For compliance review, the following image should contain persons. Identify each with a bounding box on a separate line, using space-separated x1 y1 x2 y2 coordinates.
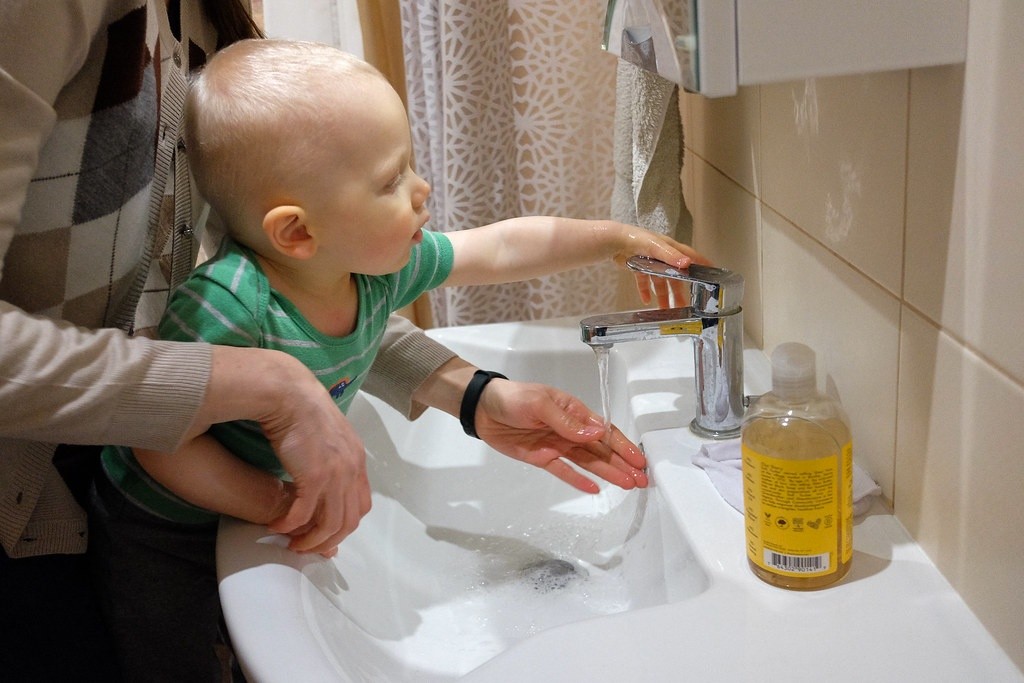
0 0 648 683
86 37 713 683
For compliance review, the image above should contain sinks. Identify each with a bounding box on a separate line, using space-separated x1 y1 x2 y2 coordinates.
213 315 1022 683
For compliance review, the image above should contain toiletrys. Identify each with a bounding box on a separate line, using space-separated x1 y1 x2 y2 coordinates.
740 342 854 591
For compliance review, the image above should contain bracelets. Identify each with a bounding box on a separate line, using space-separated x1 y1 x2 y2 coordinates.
459 369 509 441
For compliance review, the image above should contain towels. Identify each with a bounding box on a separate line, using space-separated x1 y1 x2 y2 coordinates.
599 0 694 247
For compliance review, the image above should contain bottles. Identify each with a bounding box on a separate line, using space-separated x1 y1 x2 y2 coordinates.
741 343 854 590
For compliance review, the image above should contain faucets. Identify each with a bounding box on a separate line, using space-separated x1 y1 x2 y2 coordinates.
580 256 746 438
